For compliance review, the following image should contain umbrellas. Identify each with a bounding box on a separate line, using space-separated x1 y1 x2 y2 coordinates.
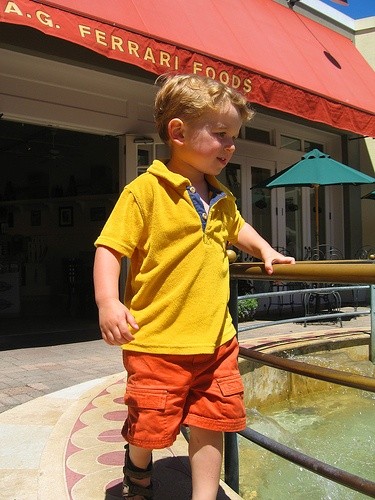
262 148 375 260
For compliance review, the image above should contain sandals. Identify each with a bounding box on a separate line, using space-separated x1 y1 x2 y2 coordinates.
122 444 154 500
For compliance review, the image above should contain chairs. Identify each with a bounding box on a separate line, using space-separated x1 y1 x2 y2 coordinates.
264 244 375 328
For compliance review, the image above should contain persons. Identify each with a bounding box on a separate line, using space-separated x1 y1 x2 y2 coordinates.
92 72 295 500
